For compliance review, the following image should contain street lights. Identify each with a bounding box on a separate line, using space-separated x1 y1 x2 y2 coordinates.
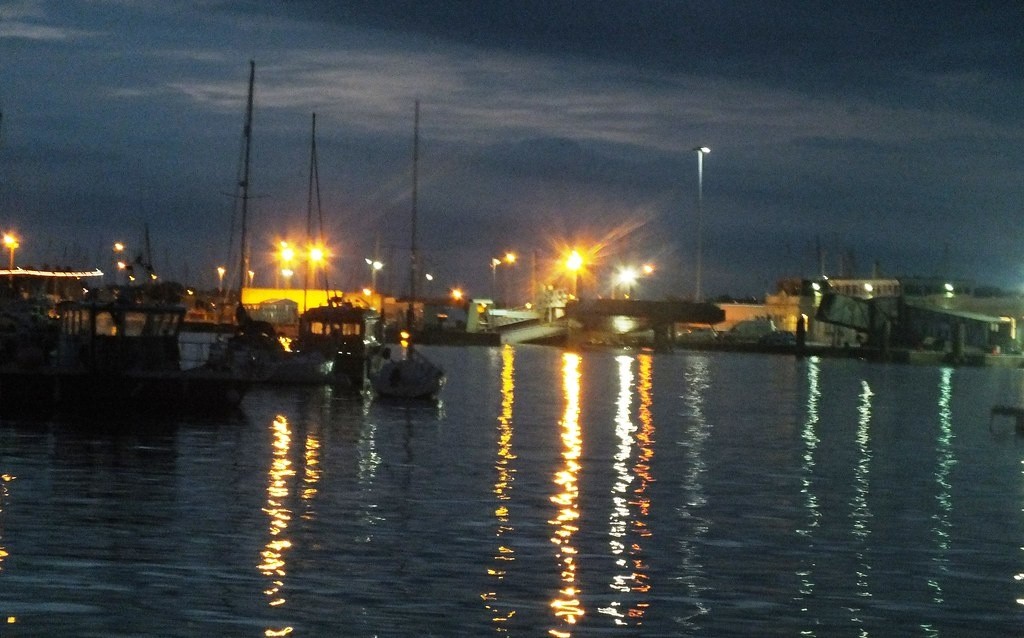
691 145 711 302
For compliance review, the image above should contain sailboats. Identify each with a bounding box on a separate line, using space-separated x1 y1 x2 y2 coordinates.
370 89 448 401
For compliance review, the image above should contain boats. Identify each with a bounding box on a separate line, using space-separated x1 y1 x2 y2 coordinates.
291 296 388 364
